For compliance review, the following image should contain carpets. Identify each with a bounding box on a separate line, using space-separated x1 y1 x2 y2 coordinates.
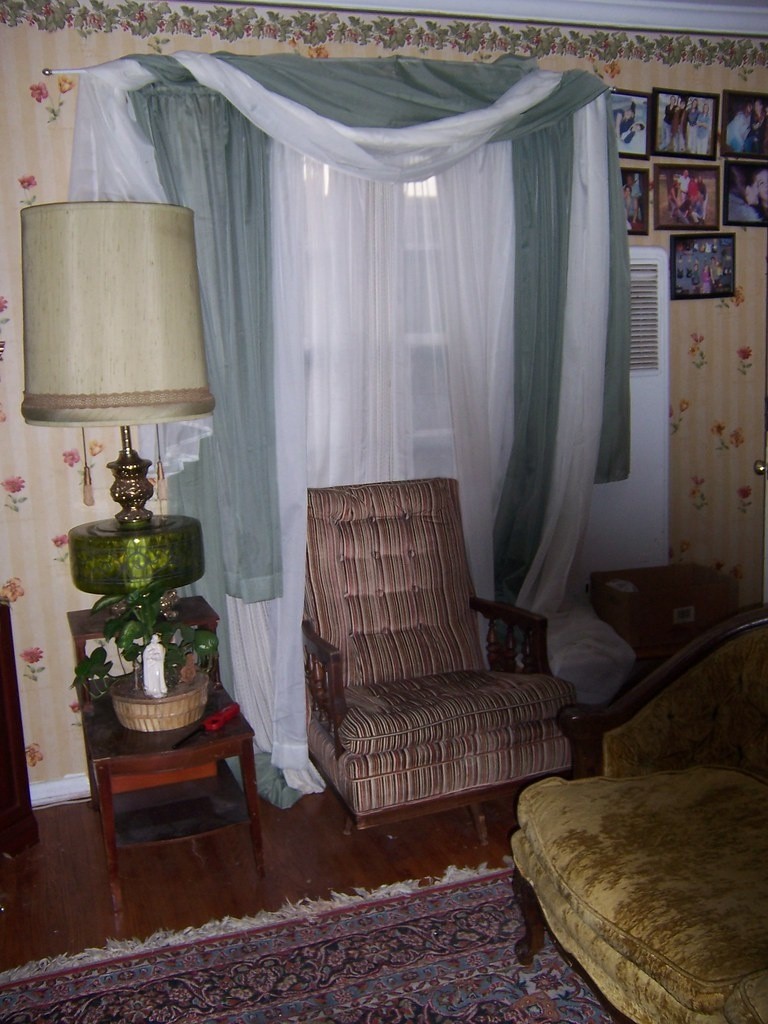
0 862 618 1024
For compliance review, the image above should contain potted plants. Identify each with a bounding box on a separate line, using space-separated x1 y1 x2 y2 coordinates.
72 571 219 733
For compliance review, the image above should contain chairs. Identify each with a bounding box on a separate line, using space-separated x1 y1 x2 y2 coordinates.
302 478 576 846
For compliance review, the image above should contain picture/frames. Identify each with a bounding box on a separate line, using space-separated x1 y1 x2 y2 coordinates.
669 232 736 300
608 86 768 236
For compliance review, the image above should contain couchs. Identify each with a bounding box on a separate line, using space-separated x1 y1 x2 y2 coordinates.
511 606 768 1024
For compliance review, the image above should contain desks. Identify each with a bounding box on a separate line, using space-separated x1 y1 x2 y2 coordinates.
63 596 265 911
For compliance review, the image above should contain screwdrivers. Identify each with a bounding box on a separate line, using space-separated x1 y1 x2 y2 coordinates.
170 701 241 751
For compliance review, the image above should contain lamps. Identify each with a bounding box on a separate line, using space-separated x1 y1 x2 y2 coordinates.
20 201 216 619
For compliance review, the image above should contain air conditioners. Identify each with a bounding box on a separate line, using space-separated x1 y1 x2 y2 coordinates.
581 245 669 579
